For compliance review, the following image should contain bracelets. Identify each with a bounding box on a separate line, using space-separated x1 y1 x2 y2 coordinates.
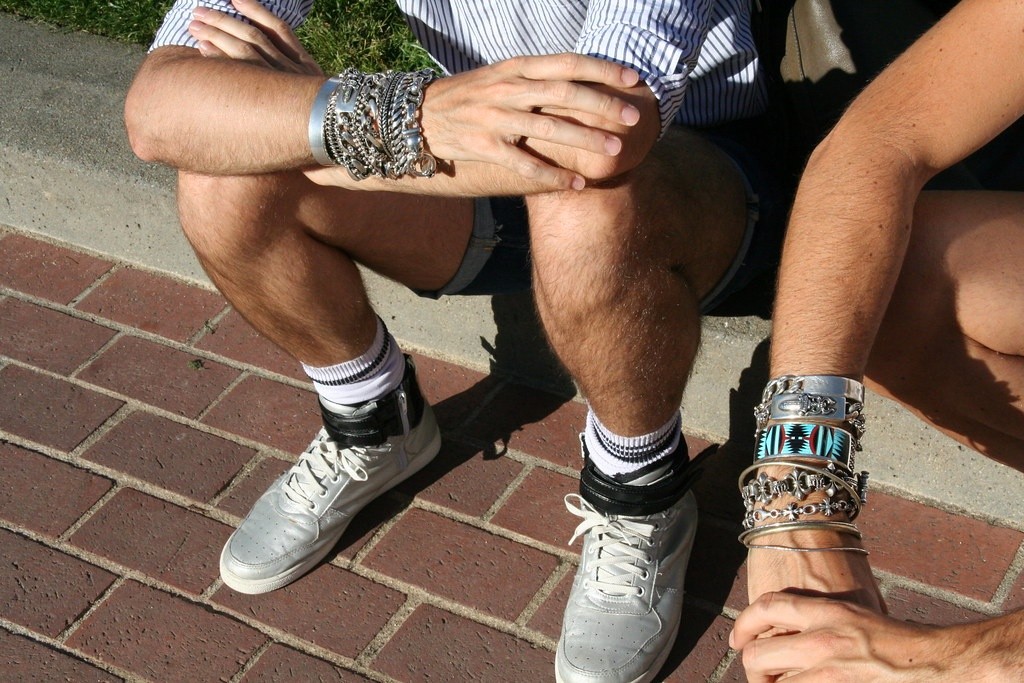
735 374 874 561
306 65 443 182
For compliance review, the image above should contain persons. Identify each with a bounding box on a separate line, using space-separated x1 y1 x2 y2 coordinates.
122 0 815 682
737 0 1023 682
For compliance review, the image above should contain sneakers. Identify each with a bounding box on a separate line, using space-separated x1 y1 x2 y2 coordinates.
220 350 442 595
554 427 719 683
